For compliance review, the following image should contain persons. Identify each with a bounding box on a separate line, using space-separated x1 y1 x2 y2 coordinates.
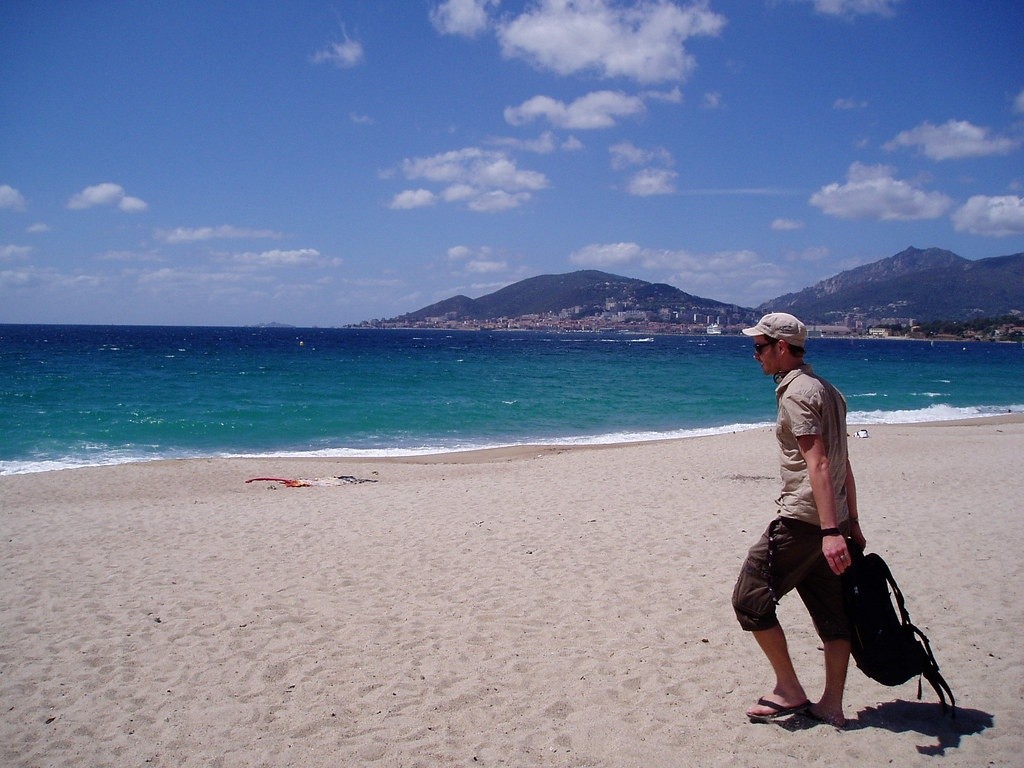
733 313 869 727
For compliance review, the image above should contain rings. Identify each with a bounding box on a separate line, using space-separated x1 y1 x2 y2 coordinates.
840 556 846 559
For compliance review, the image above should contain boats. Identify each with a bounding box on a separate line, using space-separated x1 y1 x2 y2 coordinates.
707 322 722 335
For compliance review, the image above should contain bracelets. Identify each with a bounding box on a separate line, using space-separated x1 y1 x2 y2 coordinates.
821 528 840 537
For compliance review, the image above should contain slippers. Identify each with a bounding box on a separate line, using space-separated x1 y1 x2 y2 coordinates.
793 700 849 729
746 696 810 720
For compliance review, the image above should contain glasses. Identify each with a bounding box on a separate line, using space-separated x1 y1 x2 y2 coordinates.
742 313 806 347
755 341 778 352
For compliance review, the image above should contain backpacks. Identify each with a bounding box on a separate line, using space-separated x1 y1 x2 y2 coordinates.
840 539 939 686
